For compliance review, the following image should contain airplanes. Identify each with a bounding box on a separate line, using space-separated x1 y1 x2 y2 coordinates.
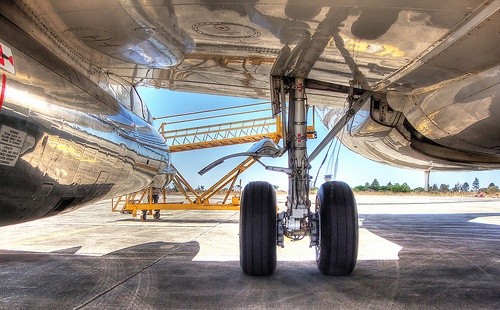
1 0 500 275
